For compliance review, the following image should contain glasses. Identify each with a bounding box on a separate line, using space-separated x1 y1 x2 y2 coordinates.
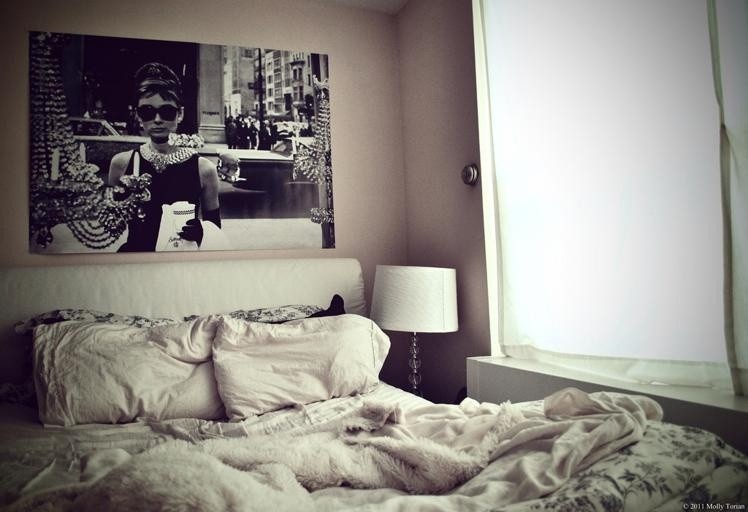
134 104 179 122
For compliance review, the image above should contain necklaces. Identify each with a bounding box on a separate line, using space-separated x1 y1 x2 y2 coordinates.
140 143 200 173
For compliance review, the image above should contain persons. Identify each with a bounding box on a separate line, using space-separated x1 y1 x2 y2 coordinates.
84 101 110 122
224 116 280 152
125 104 141 135
106 62 224 253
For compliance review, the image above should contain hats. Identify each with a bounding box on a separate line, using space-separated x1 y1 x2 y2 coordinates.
134 62 180 87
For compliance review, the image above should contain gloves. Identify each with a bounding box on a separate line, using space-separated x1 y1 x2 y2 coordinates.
178 218 203 242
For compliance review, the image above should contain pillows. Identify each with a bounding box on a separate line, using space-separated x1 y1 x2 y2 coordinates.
13 294 392 427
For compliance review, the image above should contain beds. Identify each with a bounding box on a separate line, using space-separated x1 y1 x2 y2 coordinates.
0 257 748 512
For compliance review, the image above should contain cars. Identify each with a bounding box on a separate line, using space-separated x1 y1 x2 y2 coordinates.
67 117 120 137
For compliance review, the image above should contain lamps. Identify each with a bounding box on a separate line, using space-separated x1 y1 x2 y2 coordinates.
369 264 459 397
